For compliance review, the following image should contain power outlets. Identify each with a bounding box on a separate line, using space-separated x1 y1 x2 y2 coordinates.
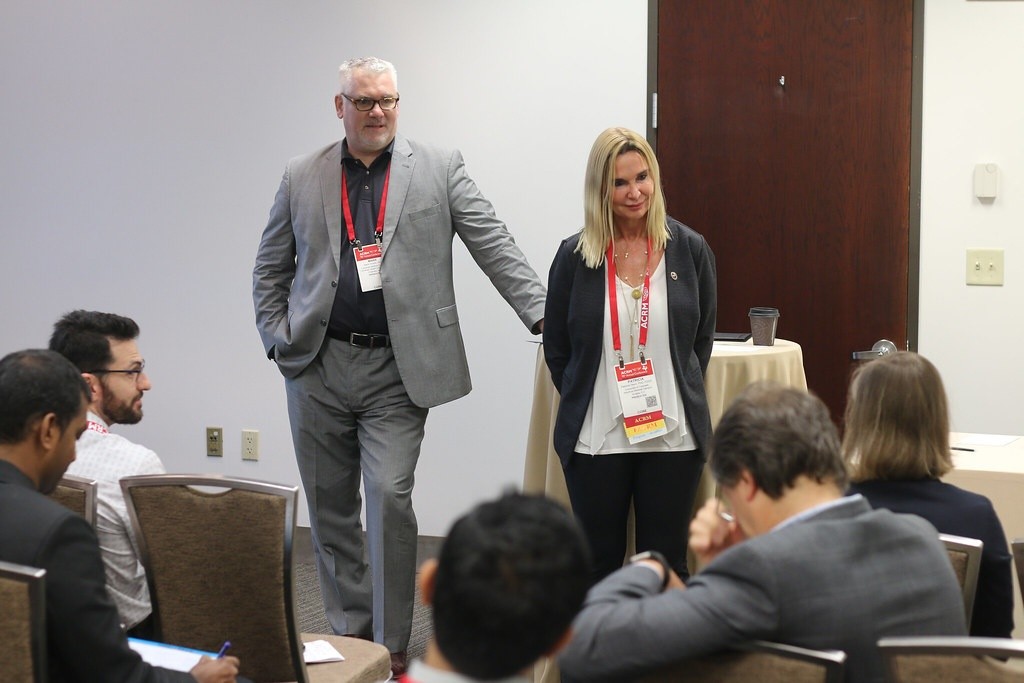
242 431 259 460
206 428 225 456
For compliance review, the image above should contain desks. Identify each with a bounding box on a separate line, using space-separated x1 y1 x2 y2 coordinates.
523 336 808 567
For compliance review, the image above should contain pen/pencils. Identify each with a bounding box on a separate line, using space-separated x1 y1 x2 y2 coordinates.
217 641 231 658
950 447 974 452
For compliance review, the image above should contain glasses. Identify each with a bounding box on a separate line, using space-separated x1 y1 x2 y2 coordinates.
341 92 400 112
87 362 145 383
714 476 742 521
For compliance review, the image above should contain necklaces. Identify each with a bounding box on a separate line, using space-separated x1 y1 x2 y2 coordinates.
614 248 648 336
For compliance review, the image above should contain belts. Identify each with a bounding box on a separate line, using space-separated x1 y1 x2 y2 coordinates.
326 327 391 348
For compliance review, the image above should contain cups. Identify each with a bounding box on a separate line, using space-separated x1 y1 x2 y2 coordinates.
748 306 780 346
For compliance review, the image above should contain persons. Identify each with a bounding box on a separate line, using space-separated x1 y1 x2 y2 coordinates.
49 309 165 635
842 350 1015 662
0 348 242 683
249 58 548 679
393 492 592 683
555 387 967 683
542 127 718 580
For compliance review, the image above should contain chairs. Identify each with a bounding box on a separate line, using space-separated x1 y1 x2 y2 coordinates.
0 470 1024 683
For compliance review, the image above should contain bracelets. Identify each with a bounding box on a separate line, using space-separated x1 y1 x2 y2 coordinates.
628 550 670 591
